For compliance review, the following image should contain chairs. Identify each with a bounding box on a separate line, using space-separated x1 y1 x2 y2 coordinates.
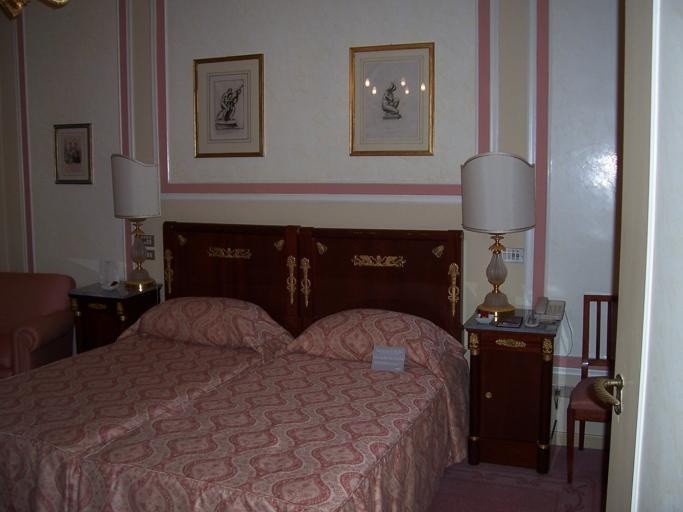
567 292 619 485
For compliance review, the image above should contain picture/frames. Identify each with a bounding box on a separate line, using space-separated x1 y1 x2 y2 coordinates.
54 122 92 185
346 42 434 156
191 54 265 159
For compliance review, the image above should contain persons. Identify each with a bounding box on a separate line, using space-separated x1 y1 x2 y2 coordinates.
216 88 240 120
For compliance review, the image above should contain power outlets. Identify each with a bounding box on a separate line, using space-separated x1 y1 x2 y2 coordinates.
548 384 574 399
152 280 164 293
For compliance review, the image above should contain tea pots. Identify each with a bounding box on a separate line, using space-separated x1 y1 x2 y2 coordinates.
99 259 118 291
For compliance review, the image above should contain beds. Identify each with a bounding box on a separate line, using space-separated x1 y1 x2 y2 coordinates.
3 221 468 512
0 224 300 510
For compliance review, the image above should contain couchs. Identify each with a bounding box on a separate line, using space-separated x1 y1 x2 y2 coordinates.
0 272 77 378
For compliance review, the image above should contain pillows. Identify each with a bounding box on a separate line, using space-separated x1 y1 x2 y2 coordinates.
286 307 465 374
120 294 296 354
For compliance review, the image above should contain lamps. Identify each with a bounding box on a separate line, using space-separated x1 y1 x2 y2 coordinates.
459 152 537 324
112 154 163 290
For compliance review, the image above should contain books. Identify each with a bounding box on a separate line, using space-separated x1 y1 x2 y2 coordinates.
496 315 523 328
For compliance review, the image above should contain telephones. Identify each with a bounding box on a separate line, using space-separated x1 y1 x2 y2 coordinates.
534 297 566 325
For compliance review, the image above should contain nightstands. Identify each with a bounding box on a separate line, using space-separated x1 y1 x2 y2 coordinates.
69 282 159 352
464 308 561 473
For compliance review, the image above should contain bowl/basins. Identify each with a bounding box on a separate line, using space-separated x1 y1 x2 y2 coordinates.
474 313 494 325
